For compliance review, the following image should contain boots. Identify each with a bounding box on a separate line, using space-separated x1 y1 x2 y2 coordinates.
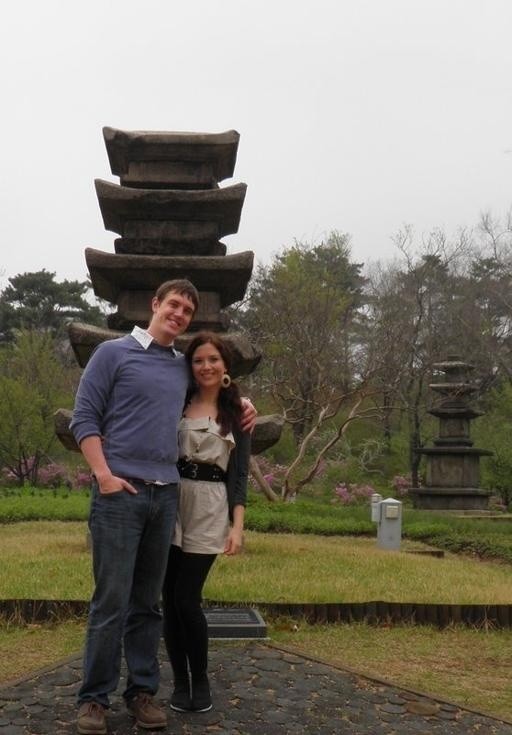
169 657 191 712
189 655 212 713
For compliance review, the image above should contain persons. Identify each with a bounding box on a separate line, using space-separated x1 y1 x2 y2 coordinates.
102 331 250 712
68 278 258 733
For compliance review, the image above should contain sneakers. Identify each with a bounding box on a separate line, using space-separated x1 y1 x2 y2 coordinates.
77 700 106 734
127 694 167 730
